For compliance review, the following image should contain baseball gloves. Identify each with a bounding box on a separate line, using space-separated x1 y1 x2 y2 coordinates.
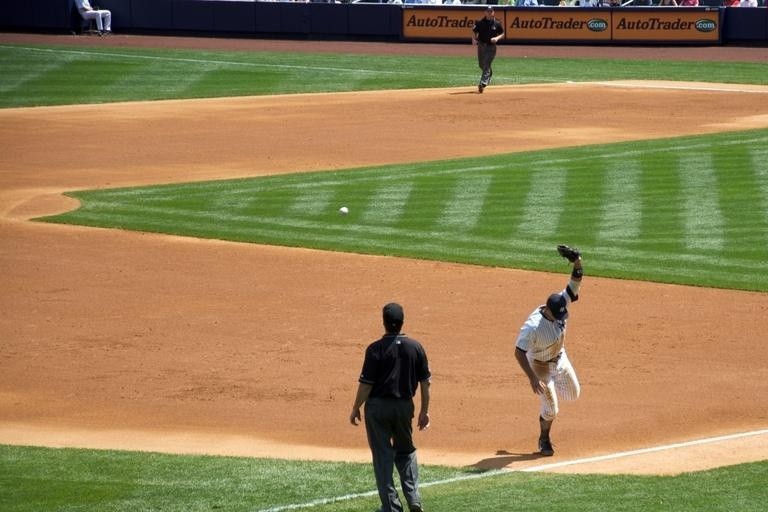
556 244 581 263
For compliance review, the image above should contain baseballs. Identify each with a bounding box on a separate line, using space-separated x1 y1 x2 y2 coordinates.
340 207 350 216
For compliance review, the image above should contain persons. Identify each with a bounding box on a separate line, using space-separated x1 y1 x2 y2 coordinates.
350 302 431 511
516 244 583 457
74 0 113 36
472 7 505 93
386 0 759 7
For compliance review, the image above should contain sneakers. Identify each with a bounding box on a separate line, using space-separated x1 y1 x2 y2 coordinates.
538 437 554 456
409 503 423 512
478 72 491 94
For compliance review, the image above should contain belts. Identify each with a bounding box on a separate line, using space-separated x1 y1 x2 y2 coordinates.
534 352 563 365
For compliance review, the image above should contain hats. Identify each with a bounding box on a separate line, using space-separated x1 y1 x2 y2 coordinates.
382 303 404 331
546 294 569 320
484 5 494 11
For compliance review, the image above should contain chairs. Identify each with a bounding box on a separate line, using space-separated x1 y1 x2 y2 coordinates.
73 0 102 36
